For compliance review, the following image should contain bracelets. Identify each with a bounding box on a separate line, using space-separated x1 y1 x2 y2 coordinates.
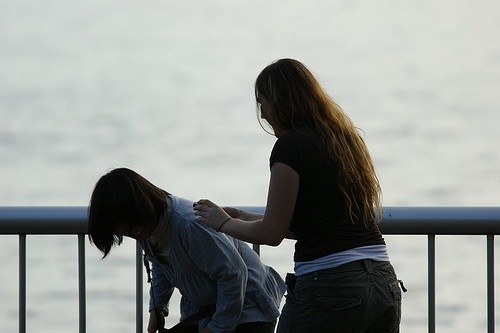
216 218 231 232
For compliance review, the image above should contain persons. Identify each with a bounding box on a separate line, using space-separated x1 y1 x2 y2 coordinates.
192 58 407 332
87 167 287 333
